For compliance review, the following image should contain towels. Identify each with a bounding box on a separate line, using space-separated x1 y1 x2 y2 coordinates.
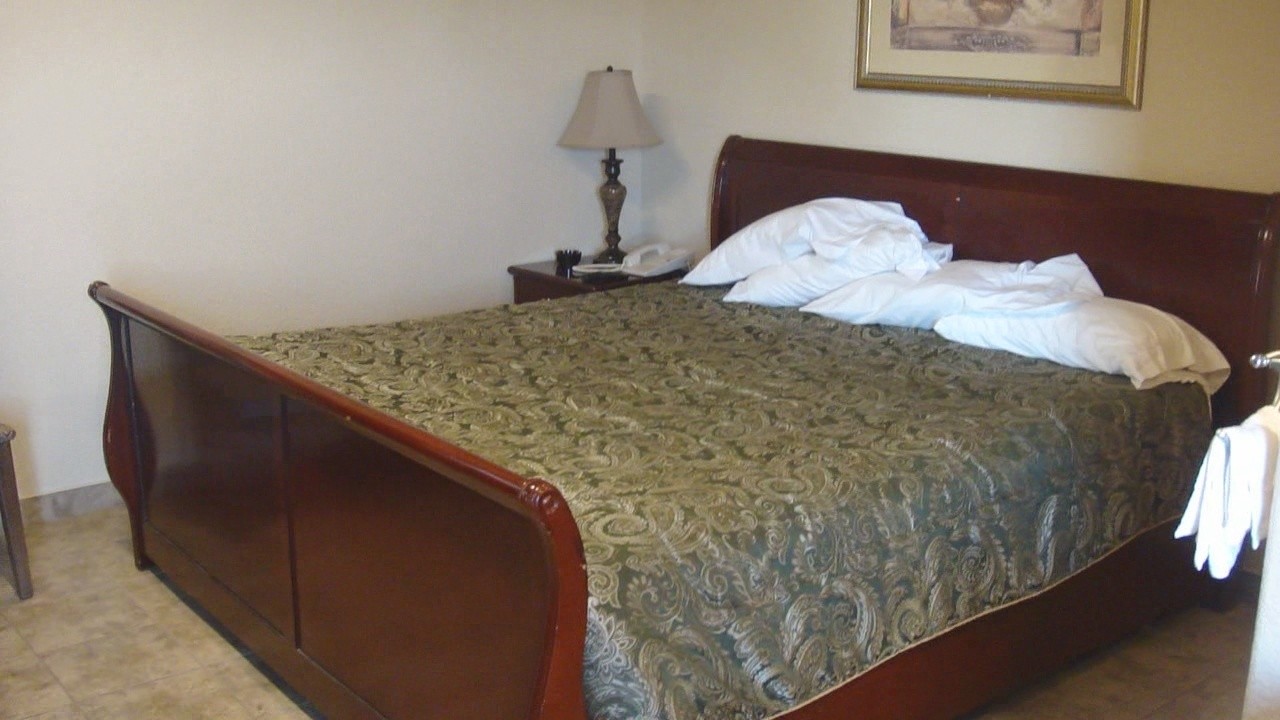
1173 424 1266 580
1243 404 1280 552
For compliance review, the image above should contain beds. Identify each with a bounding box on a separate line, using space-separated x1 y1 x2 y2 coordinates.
86 134 1280 720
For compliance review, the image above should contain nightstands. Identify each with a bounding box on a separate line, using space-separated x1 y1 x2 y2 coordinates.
508 256 684 303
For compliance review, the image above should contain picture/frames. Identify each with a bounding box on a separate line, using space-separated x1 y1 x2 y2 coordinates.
853 0 1151 111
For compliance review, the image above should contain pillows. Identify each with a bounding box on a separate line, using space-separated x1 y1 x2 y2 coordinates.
677 195 1233 394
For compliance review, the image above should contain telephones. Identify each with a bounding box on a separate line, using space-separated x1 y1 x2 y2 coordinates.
620 244 697 278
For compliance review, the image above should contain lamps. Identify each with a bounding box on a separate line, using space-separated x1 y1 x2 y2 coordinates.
555 65 666 263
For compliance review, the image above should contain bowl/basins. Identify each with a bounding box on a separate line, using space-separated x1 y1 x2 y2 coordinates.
557 250 581 267
